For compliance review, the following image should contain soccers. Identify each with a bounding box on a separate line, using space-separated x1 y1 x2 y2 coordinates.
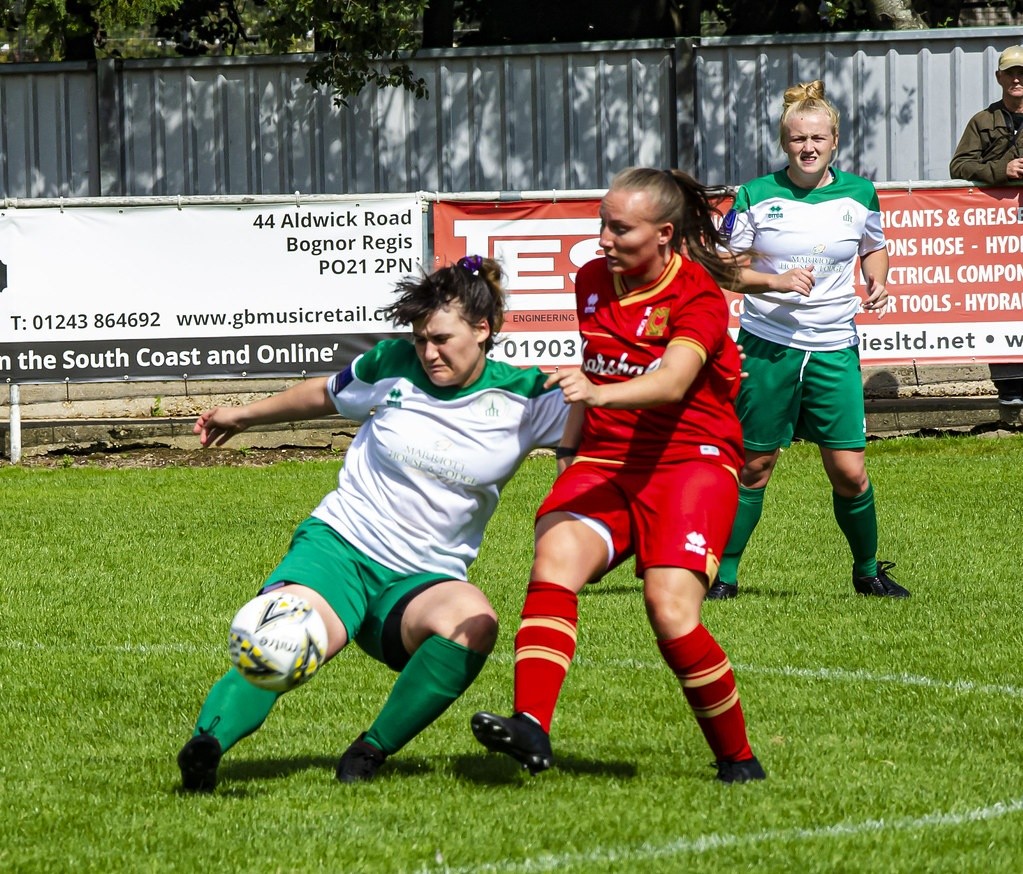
228 591 329 693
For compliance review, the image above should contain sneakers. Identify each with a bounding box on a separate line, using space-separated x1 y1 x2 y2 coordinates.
471 711 553 774
852 560 911 598
336 732 389 786
703 574 738 601
177 715 221 794
710 756 766 785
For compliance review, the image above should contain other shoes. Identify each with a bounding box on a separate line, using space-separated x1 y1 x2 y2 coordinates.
997 389 1023 405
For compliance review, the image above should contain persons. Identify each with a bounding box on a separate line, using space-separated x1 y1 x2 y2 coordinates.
177 258 573 794
710 78 915 598
948 45 1023 432
471 167 767 785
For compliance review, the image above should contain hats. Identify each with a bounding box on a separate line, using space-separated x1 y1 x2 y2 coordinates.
998 44 1023 71
